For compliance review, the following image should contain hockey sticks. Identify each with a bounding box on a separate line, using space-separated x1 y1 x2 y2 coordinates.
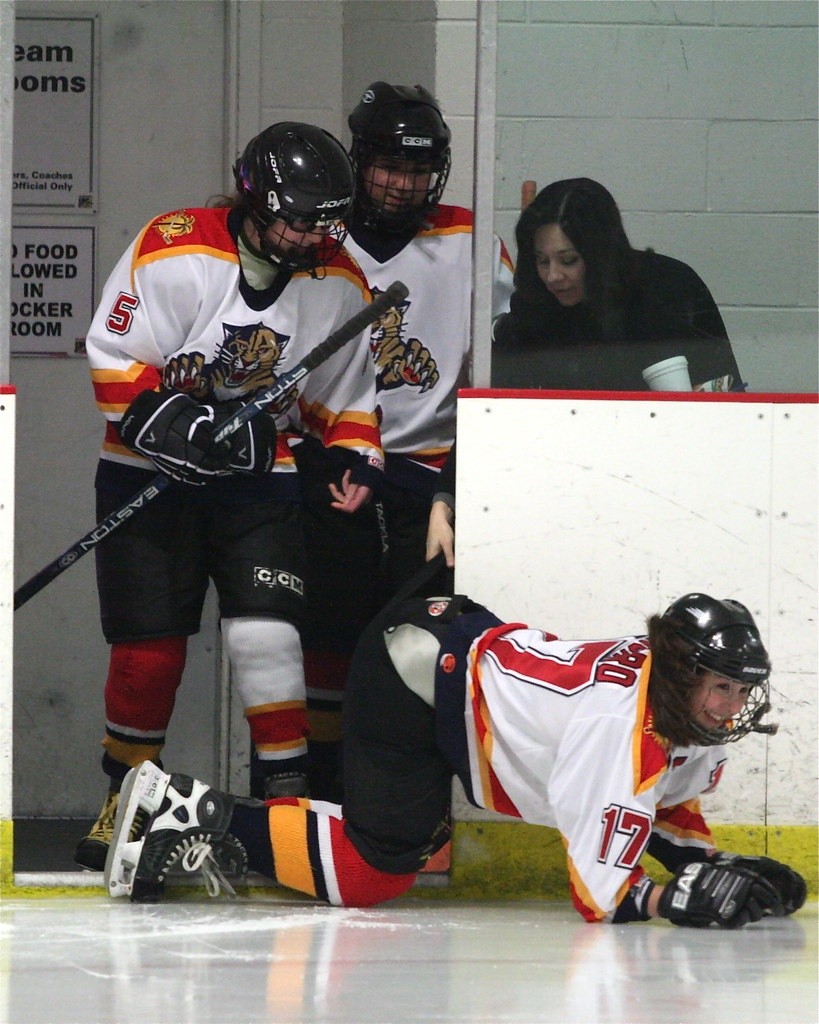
14 275 412 617
515 179 538 269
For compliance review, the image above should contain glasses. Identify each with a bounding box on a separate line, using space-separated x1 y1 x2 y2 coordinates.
265 206 344 233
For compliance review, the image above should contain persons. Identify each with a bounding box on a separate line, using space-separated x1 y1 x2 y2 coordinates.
77 122 385 874
128 593 808 929
426 179 742 599
305 83 515 806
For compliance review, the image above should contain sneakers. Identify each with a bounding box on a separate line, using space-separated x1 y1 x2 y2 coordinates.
103 761 248 901
74 779 125 873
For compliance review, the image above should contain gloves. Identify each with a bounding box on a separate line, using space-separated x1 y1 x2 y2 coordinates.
709 848 807 915
658 863 784 929
116 384 236 488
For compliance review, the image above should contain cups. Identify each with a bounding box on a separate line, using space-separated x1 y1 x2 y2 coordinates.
642 355 693 391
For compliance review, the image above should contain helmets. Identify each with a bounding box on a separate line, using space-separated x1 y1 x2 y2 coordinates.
662 593 771 746
237 123 359 272
348 80 453 228
